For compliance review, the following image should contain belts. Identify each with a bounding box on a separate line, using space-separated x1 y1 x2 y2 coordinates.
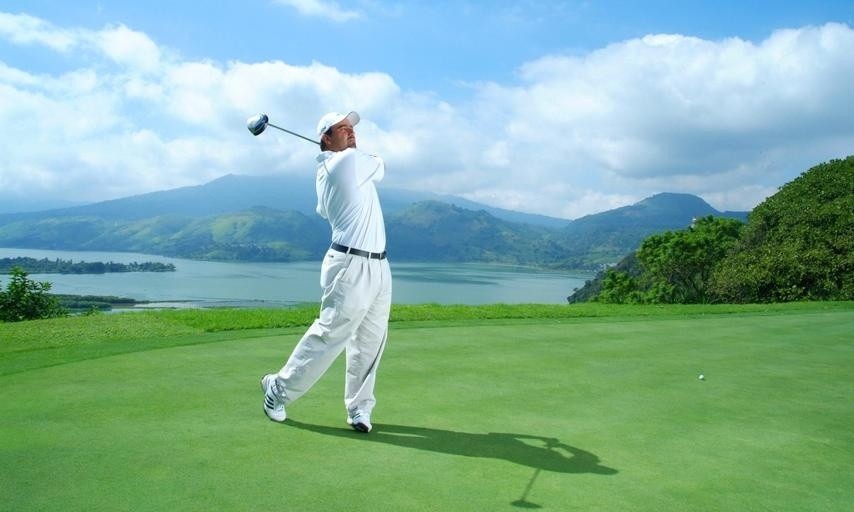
330 243 387 260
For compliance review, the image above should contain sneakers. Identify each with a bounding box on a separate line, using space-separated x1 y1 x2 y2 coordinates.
347 410 373 433
260 373 287 423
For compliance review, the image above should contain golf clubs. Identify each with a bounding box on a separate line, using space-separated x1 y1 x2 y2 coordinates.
247 114 321 146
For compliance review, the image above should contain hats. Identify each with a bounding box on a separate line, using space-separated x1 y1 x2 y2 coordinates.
317 110 361 135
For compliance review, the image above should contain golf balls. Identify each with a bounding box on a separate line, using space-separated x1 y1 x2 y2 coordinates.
699 375 704 379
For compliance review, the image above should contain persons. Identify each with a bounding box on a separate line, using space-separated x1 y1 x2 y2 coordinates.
261 111 393 432
